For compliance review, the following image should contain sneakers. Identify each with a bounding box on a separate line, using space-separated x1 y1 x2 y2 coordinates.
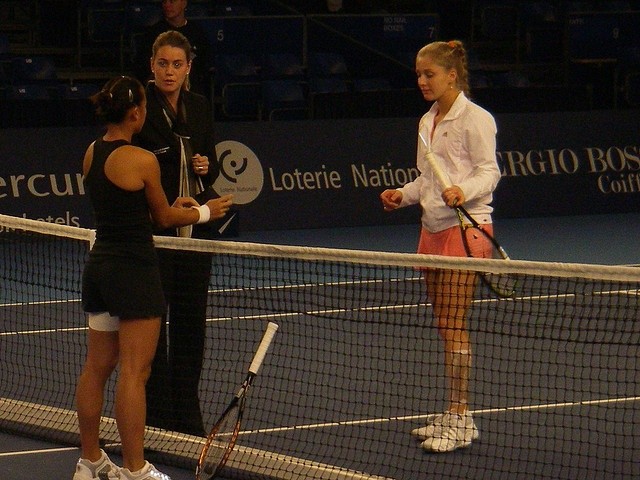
72 448 120 479
107 460 171 480
420 411 471 454
410 410 480 440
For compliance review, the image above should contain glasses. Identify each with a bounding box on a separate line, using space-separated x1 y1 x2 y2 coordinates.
150 58 188 68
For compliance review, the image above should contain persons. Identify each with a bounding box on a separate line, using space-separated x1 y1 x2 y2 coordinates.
141 1 213 76
381 39 501 454
141 28 221 437
74 76 235 480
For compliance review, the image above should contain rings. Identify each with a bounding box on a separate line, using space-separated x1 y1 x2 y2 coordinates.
198 166 203 172
